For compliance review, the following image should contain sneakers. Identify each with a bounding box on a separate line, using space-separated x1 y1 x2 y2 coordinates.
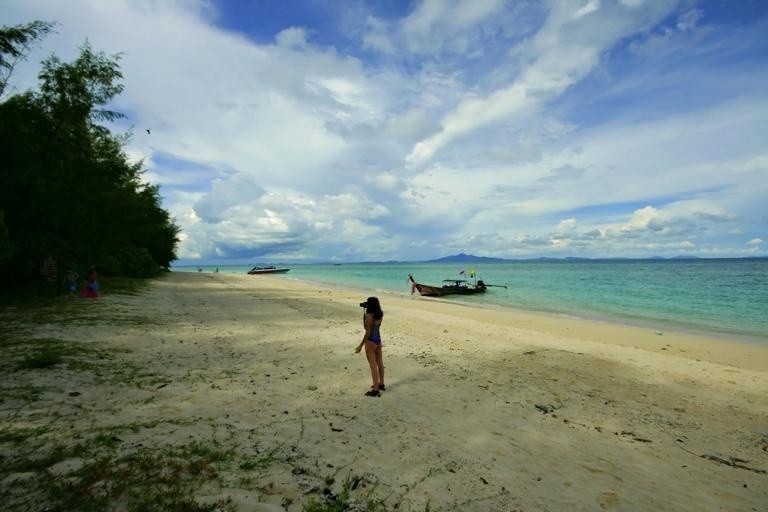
365 390 380 397
371 383 385 391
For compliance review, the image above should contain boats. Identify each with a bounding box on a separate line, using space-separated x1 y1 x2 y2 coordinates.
407 273 487 296
248 265 291 275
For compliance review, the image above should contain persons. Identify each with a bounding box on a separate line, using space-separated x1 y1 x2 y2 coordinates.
354 297 385 397
63 264 79 293
87 264 97 291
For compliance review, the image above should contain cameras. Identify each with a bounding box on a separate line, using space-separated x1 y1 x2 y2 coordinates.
360 302 368 308
